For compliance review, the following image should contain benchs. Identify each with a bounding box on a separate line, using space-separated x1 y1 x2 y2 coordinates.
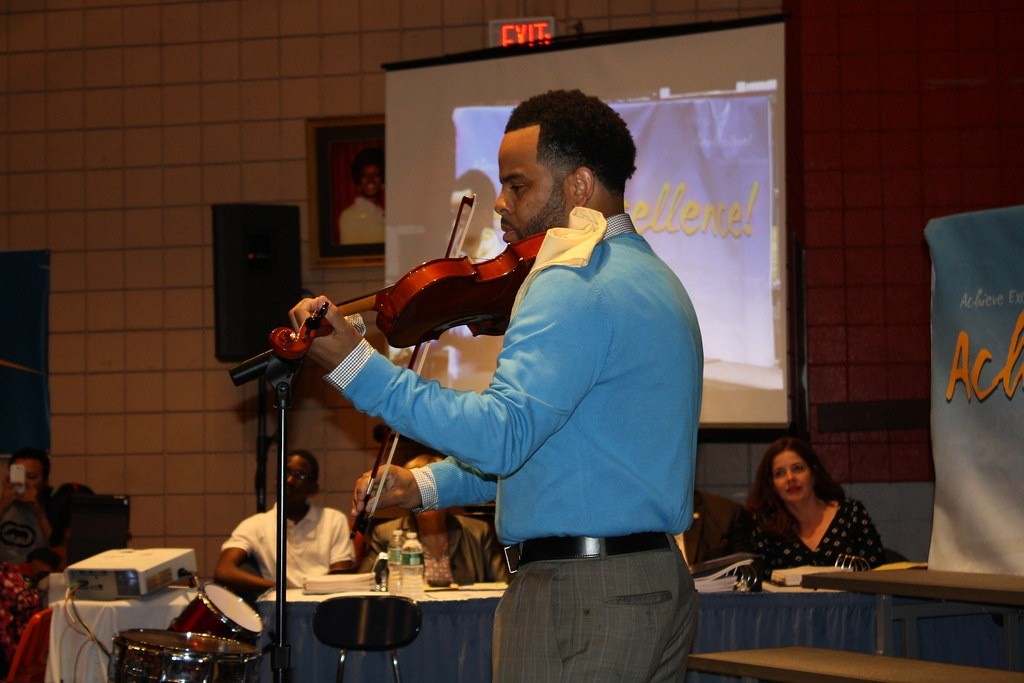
688 646 1024 683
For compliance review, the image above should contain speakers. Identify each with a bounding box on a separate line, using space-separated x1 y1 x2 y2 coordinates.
212 202 303 363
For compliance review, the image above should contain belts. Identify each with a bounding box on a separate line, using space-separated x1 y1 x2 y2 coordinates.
519 532 670 566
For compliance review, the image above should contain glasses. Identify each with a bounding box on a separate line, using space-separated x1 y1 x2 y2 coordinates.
286 470 314 484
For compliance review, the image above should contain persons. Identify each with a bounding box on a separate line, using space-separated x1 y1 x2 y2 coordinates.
218 449 511 602
390 169 512 394
1 450 92 683
290 89 706 682
338 148 385 243
741 436 887 570
674 485 752 580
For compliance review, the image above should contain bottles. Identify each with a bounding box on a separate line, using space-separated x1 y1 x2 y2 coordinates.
386 530 405 594
401 532 424 600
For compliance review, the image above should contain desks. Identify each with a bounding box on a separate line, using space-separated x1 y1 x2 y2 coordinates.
802 568 1024 672
257 580 846 683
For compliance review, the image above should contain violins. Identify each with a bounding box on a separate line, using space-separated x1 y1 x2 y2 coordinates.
268 231 552 362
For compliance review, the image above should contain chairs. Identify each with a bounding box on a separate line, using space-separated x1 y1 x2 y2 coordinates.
6 608 53 683
313 595 420 683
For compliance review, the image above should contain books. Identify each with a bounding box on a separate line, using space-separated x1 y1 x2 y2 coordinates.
694 558 928 593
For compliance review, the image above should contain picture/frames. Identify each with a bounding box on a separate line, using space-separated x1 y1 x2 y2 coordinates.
306 115 385 265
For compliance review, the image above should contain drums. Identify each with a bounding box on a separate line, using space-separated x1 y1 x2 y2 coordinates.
168 581 264 643
106 627 262 683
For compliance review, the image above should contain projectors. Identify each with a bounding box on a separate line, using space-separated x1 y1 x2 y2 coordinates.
65 548 197 601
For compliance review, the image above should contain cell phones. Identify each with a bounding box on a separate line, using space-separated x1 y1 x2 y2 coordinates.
10 464 25 493
